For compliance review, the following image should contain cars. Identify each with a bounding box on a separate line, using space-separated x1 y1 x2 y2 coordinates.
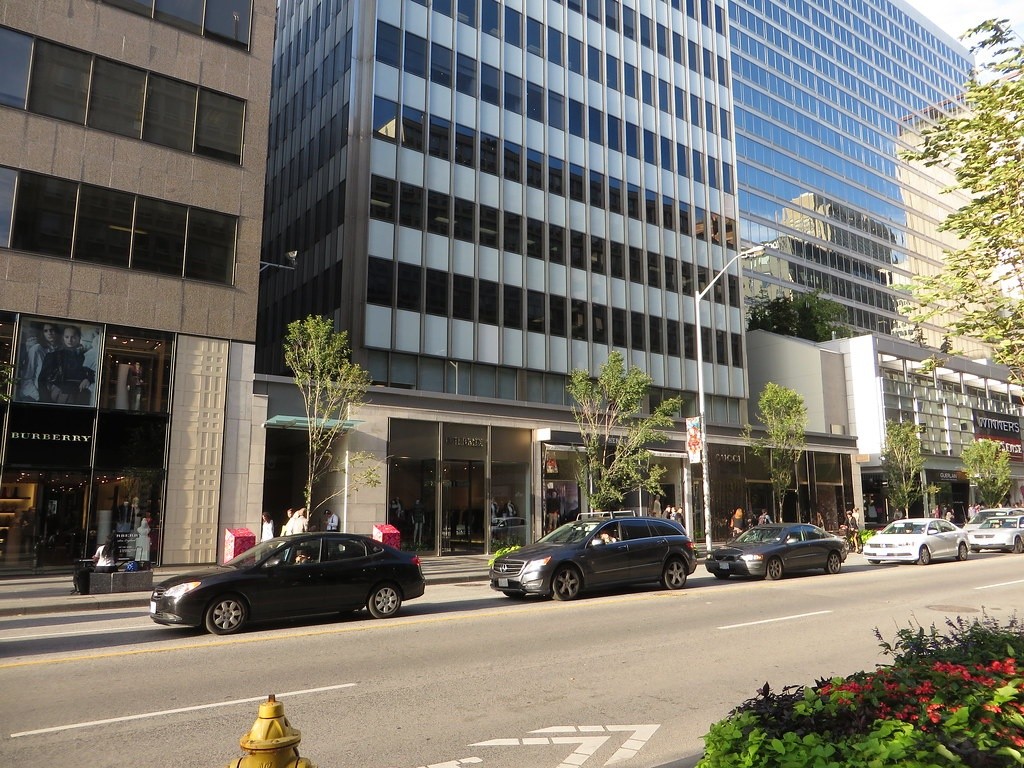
149 532 426 635
490 517 527 538
704 523 847 580
961 508 1024 531
862 518 971 565
490 510 699 601
967 515 1024 554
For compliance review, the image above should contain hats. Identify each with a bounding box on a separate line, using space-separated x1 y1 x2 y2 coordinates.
297 549 315 561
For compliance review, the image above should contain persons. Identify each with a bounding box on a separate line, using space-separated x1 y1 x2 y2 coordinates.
325 509 341 553
92 533 118 573
546 485 561 533
284 508 309 563
491 497 518 547
412 499 426 549
297 548 316 564
893 508 903 520
21 322 93 406
731 508 745 539
601 528 616 544
758 509 773 539
654 495 662 518
127 362 145 412
776 533 797 543
841 507 861 553
968 500 1024 529
934 504 955 524
260 513 273 565
113 497 149 560
664 505 686 528
389 497 401 527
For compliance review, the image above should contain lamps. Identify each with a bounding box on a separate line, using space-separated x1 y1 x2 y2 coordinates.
258 249 298 272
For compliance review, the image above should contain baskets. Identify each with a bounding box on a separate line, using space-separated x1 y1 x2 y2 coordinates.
839 527 848 536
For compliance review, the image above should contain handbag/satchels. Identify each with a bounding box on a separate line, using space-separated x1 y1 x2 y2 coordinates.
764 514 769 524
124 560 138 572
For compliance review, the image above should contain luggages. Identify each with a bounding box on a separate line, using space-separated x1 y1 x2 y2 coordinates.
76 567 94 595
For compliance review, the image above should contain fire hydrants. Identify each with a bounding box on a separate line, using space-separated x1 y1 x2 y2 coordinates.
224 694 319 768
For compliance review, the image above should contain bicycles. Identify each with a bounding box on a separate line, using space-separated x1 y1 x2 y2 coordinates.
842 527 863 554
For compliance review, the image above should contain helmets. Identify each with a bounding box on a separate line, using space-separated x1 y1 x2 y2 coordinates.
846 511 852 515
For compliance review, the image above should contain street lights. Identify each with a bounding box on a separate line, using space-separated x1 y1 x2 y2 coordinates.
695 245 766 559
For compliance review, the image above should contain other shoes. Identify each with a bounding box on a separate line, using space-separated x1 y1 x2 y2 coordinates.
855 547 859 553
